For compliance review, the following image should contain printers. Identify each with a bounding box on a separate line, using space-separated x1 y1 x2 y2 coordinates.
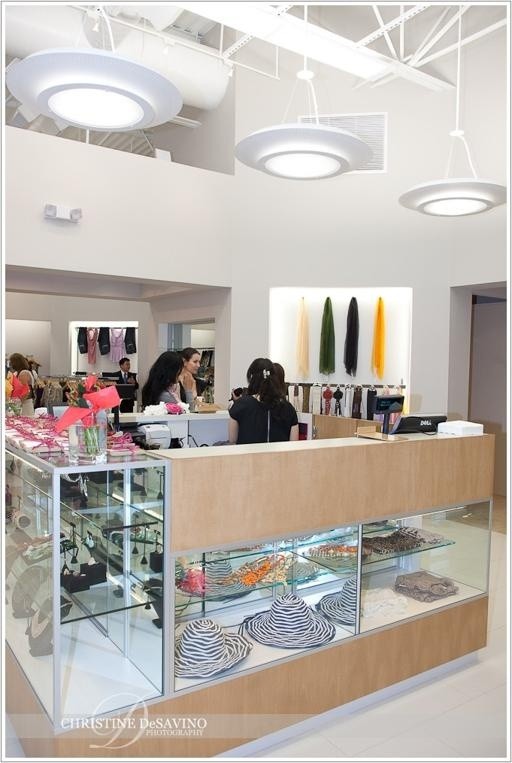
141 423 173 448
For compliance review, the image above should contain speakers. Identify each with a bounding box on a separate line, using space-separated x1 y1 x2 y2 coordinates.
43 203 84 223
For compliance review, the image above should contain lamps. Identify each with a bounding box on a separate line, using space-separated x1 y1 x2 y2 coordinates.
397 5 506 216
6 6 182 132
233 6 372 180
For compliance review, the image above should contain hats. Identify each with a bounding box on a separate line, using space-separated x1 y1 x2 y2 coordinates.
205 557 255 601
278 562 318 584
175 594 336 678
318 576 356 626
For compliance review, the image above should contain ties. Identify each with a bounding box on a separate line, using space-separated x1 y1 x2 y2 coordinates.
124 373 126 384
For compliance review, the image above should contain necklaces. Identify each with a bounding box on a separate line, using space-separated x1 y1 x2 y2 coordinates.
31 602 72 638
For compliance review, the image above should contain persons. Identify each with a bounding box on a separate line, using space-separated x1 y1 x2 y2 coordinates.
228 358 299 444
142 347 201 411
112 358 139 413
11 353 41 399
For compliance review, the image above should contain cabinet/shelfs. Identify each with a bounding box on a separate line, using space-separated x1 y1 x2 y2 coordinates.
5 418 492 758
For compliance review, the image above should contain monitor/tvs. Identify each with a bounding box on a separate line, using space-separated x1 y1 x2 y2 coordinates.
391 413 447 434
